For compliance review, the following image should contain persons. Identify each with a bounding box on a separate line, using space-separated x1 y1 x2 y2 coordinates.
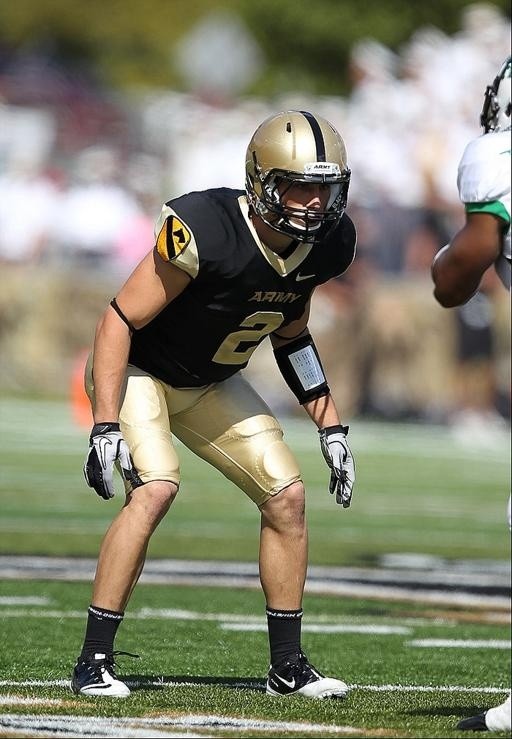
71 111 356 703
427 57 512 734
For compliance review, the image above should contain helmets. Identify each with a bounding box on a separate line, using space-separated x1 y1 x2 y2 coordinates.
244 108 352 243
480 53 512 133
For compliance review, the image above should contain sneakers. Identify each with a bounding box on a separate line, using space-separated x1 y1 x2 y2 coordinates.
70 648 131 700
265 650 351 701
456 693 512 733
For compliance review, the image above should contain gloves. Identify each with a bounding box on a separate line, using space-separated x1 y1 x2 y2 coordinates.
317 424 357 510
81 420 134 501
